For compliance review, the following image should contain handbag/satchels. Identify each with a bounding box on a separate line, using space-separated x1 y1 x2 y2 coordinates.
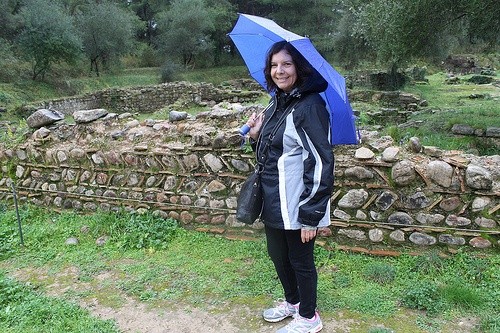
236 171 263 224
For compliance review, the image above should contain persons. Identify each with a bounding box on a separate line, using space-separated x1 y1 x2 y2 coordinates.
245 40 335 333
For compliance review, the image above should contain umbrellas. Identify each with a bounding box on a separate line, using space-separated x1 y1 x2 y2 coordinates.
226 13 361 147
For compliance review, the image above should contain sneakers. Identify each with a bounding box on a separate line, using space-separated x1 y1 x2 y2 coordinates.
276 312 323 333
262 298 300 322
